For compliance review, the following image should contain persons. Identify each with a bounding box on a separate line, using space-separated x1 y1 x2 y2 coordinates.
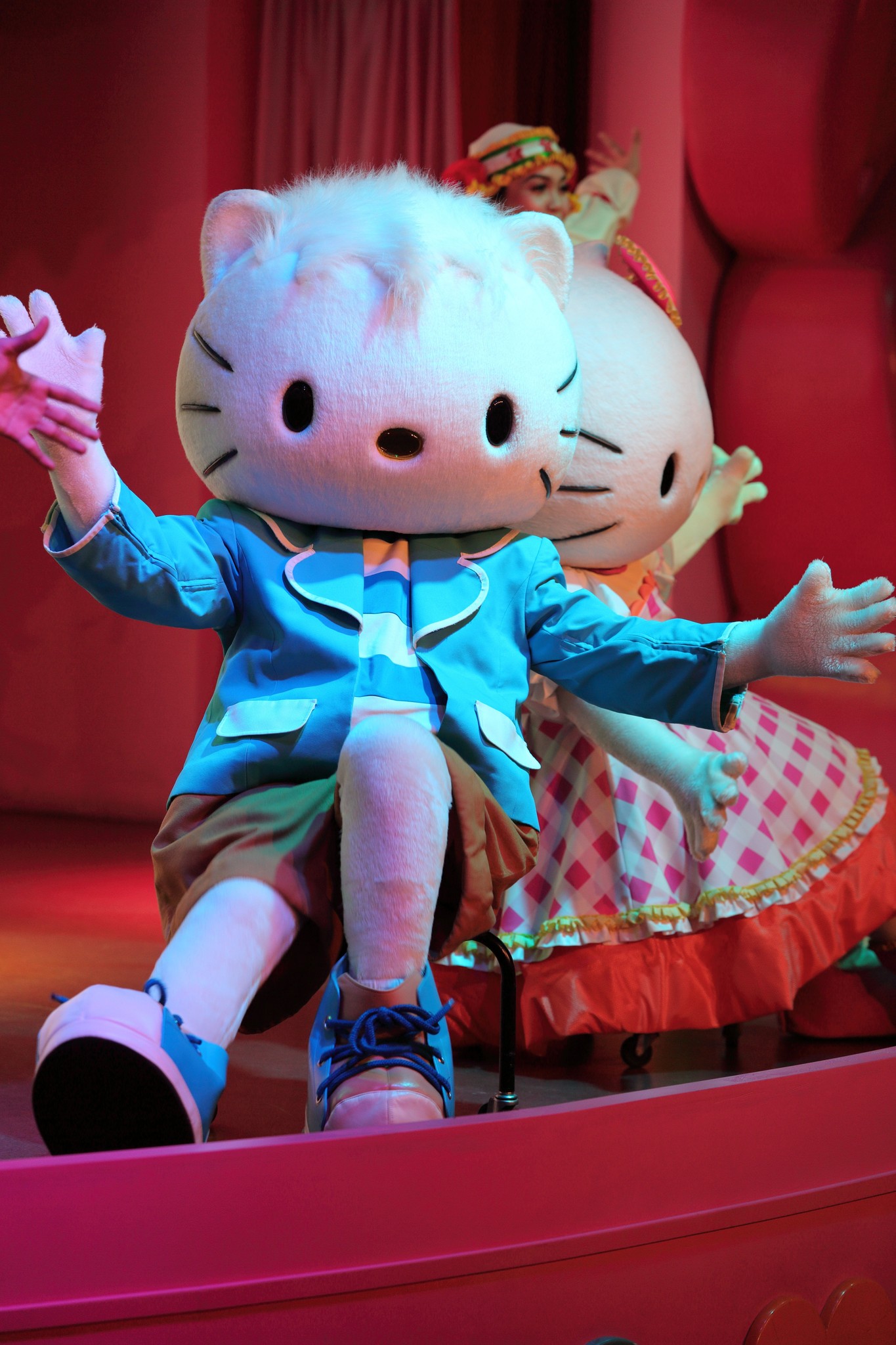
1 316 103 468
441 123 645 267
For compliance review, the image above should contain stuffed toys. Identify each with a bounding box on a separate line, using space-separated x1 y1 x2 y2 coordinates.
436 264 891 1094
0 162 895 1158
